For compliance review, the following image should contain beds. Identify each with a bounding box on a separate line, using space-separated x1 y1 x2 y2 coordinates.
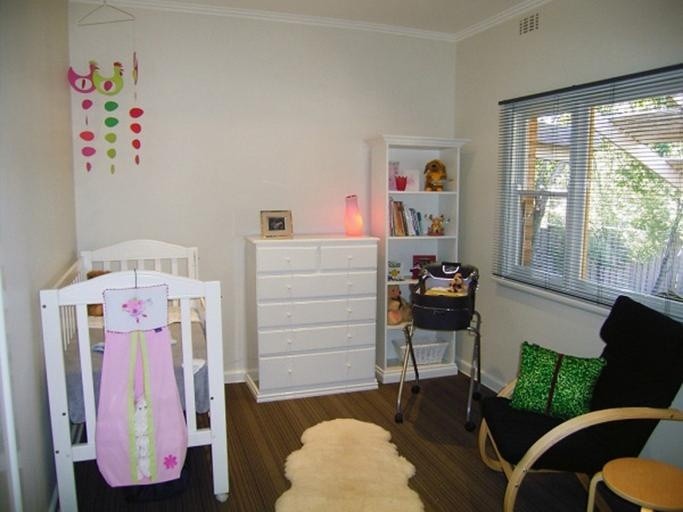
37 237 232 512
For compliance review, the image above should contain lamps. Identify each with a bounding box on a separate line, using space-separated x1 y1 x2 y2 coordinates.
343 194 363 236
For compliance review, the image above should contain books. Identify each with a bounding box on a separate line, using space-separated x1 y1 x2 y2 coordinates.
389 196 424 237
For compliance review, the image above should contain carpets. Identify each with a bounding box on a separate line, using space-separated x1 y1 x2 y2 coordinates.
269 417 427 512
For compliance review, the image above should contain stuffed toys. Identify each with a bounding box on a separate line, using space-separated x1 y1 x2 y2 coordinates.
447 272 467 292
387 285 413 326
422 158 447 192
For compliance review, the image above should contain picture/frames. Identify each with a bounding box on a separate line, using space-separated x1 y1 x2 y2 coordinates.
411 255 436 278
259 208 294 239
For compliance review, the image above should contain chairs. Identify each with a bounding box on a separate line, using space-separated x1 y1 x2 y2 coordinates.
475 294 682 512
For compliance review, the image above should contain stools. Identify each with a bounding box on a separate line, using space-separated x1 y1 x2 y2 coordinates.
585 457 683 512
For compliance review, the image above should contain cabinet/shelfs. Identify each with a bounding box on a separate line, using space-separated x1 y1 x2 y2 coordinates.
364 133 467 387
244 232 379 404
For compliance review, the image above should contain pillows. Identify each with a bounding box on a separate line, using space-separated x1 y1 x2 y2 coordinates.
508 339 607 422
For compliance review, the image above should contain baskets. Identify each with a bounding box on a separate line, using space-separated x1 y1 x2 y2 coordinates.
393 338 450 365
